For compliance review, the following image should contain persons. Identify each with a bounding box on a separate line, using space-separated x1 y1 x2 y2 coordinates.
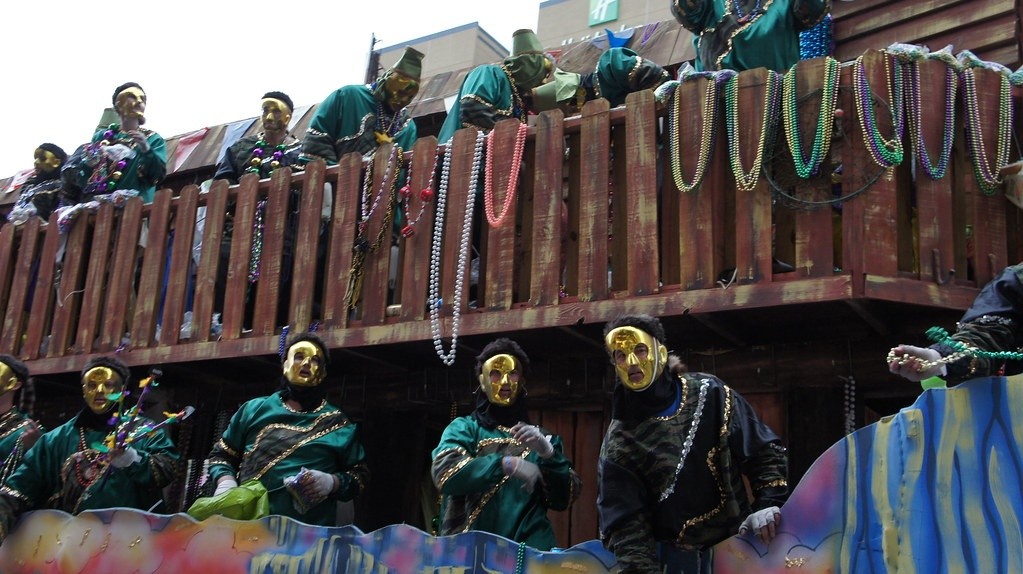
431 337 582 551
529 48 671 114
210 334 374 523
215 89 301 283
887 263 1022 387
669 1 832 73
7 143 68 227
298 47 426 250
0 356 48 488
0 357 185 549
60 81 168 249
437 31 555 198
597 315 789 574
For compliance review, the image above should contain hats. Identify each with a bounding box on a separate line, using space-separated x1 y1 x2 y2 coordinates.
512 29 544 55
394 46 424 83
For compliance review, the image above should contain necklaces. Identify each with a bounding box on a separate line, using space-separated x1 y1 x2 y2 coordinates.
670 47 1013 195
339 120 528 366
247 136 285 177
87 123 128 191
249 199 267 282
0 424 44 488
515 542 525 573
374 93 399 139
77 426 113 487
888 327 1022 370
